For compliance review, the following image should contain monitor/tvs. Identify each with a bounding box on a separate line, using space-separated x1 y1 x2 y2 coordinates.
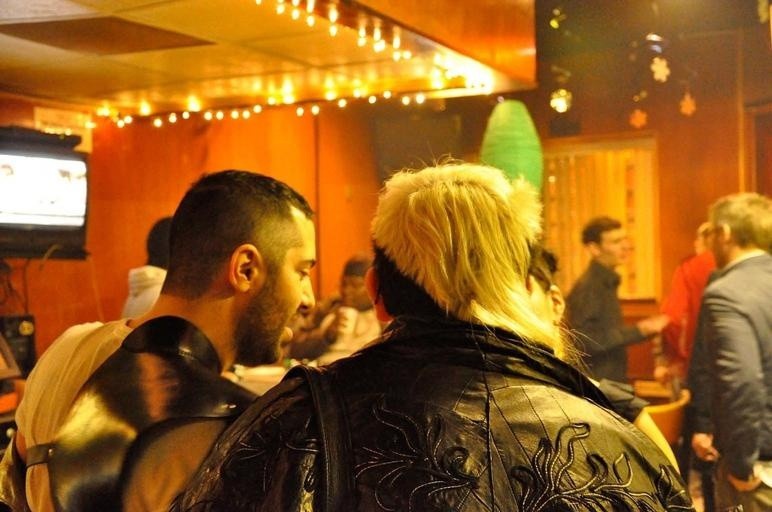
0 139 89 230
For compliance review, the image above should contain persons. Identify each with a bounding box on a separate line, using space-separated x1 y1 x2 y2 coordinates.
1 168 320 512
525 244 567 328
698 187 772 512
283 253 393 369
678 263 731 511
563 216 671 382
513 244 685 495
122 216 174 320
659 221 719 376
165 155 697 512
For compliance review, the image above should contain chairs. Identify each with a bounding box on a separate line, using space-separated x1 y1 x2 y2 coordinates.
645 387 691 478
